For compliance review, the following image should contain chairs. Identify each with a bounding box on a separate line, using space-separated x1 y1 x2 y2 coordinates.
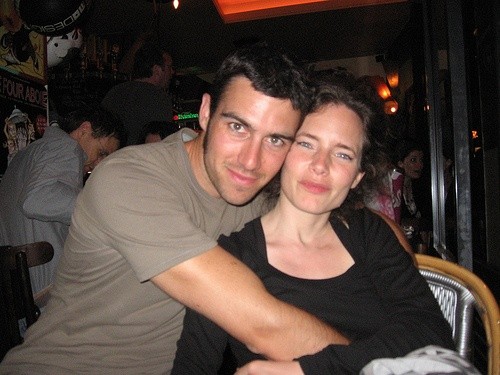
415 253 500 375
0 241 54 364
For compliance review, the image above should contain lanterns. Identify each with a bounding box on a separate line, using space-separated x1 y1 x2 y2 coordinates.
376 71 399 115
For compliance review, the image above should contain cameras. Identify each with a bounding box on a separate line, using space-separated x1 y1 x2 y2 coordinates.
403 226 415 239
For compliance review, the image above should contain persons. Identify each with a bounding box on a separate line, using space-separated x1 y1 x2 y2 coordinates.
0 41 477 337
171 68 459 375
0 43 353 375
